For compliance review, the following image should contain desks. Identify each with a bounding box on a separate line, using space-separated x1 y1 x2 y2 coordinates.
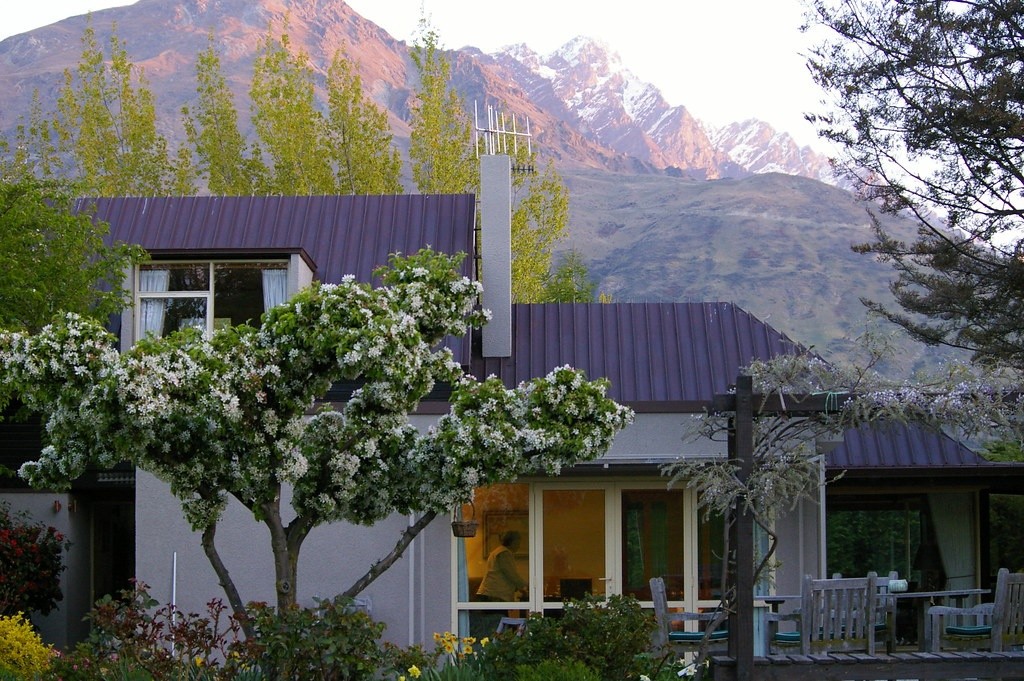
754 588 991 652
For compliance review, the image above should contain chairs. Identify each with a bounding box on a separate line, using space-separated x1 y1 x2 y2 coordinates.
648 571 901 659
927 568 1024 652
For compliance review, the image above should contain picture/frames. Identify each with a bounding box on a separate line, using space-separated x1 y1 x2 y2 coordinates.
482 509 529 559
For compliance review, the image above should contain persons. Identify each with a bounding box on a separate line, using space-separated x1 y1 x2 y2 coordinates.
475 531 528 637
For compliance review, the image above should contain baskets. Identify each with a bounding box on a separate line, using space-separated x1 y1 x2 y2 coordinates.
451 499 480 538
